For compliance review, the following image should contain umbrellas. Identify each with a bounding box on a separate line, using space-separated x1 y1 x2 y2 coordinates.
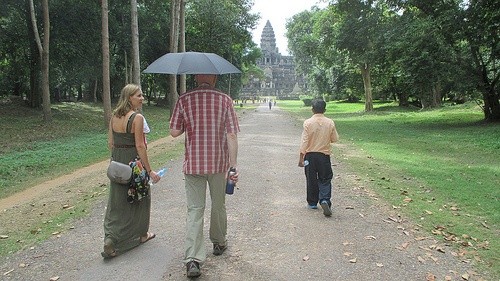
143 50 243 75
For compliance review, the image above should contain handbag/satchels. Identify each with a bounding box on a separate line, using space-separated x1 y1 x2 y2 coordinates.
107 161 133 184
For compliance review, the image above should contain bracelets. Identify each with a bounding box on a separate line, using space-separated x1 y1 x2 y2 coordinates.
147 169 151 172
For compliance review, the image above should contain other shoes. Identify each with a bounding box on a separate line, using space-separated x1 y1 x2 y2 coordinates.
310 204 317 209
213 242 226 255
321 201 331 215
187 261 201 276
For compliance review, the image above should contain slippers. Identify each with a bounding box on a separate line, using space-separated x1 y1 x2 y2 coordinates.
101 244 114 259
139 233 157 243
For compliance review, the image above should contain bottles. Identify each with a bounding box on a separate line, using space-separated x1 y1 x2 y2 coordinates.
150 168 167 183
302 160 309 166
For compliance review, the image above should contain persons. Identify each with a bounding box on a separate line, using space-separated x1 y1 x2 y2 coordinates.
298 99 339 216
169 73 241 277
234 98 277 111
101 84 157 258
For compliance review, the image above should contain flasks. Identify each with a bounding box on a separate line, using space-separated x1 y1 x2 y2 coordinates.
226 166 236 194
